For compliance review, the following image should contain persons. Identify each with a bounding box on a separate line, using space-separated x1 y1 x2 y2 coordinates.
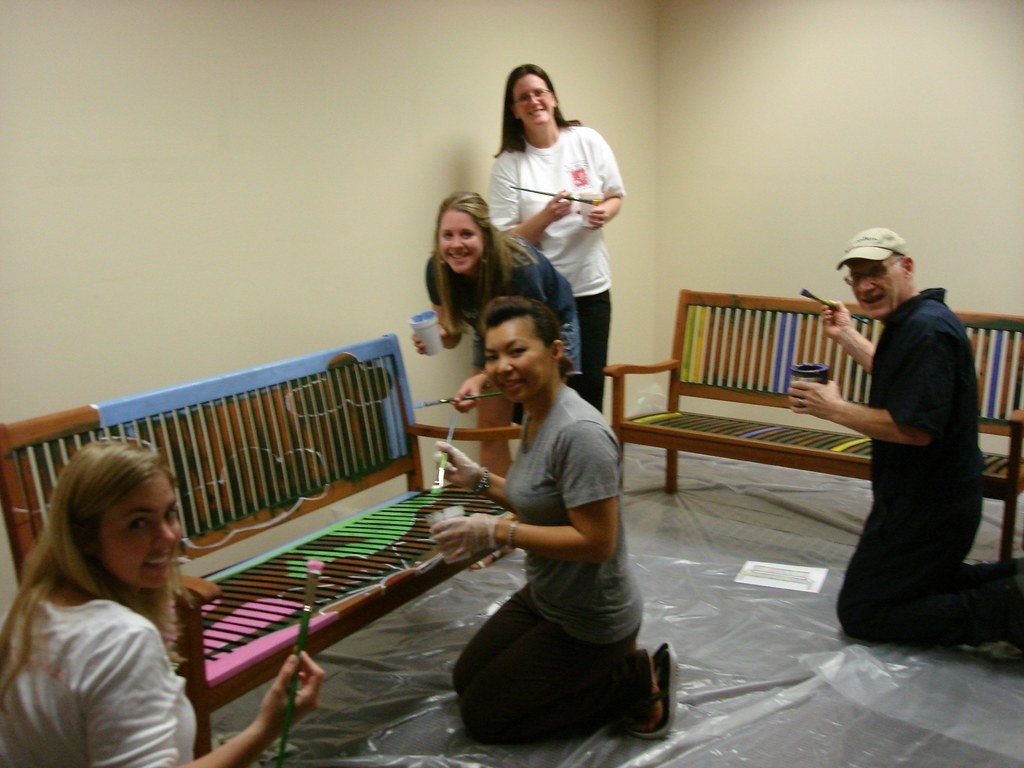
1 440 325 767
787 228 1024 651
411 63 679 746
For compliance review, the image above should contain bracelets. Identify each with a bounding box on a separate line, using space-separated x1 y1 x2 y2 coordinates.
480 369 491 391
474 467 489 494
508 521 519 549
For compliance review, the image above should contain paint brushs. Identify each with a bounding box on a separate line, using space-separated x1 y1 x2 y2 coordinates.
274 559 325 768
415 391 503 408
510 185 603 206
799 288 872 326
430 413 457 488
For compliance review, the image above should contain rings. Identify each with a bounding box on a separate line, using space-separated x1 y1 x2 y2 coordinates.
799 399 805 408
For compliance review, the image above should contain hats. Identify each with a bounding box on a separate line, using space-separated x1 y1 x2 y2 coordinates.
838 228 906 272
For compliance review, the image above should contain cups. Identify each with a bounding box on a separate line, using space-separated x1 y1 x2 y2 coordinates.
790 363 830 385
425 504 471 564
579 192 603 227
409 311 443 356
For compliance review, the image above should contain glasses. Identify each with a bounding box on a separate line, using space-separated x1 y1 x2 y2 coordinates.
842 256 901 286
514 88 550 107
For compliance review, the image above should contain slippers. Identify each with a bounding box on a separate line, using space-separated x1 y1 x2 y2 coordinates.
630 641 677 738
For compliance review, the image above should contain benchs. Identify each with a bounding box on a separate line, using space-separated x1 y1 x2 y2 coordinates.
604 286 1024 564
1 333 523 762
